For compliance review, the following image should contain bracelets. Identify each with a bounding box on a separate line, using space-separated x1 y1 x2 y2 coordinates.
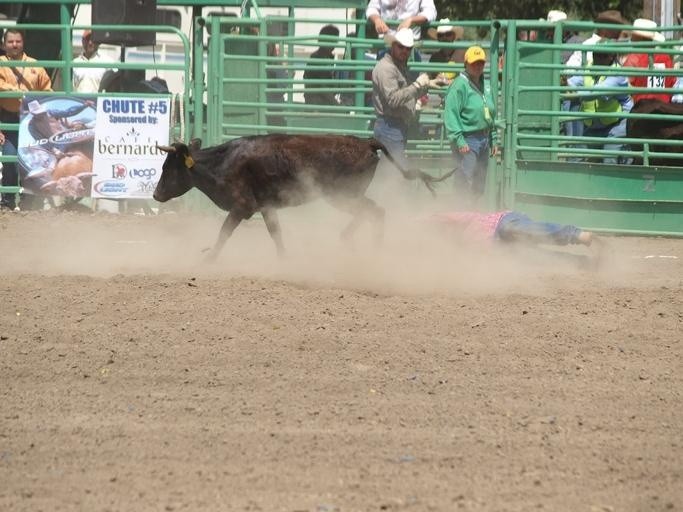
413 81 422 89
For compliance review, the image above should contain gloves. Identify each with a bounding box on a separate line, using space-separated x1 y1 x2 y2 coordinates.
412 72 430 90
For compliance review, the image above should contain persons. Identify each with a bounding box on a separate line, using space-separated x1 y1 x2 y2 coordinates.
302 24 341 106
444 46 497 208
427 18 484 140
365 1 438 61
422 209 609 276
369 27 450 200
27 96 95 160
74 29 109 93
0 29 56 209
543 9 682 164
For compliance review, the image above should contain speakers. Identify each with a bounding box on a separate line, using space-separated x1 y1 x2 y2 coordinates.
91 0 156 46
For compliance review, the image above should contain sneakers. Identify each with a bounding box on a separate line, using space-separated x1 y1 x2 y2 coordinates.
578 231 592 245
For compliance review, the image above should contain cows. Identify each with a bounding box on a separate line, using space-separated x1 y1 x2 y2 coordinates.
617 99 683 167
39 146 97 203
152 131 458 267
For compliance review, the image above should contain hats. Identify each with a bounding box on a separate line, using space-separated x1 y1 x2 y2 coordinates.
27 100 46 115
547 9 566 24
464 46 486 65
437 18 453 34
382 28 415 48
624 19 665 43
593 10 627 24
82 28 93 38
